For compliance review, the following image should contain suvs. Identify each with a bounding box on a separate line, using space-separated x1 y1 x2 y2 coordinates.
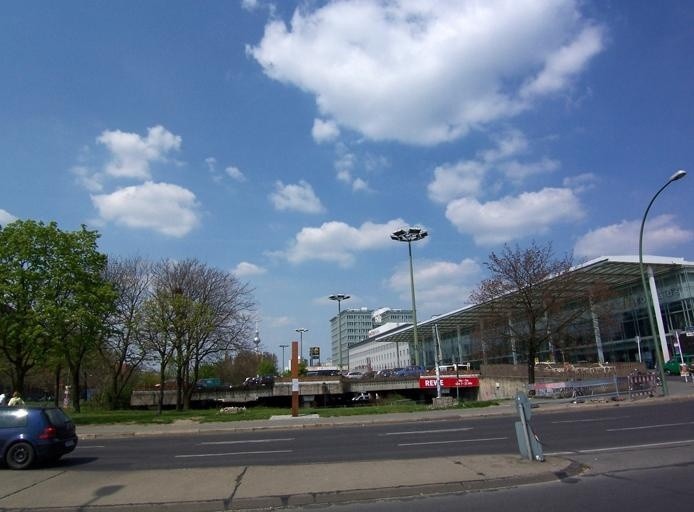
195 378 233 390
344 363 472 380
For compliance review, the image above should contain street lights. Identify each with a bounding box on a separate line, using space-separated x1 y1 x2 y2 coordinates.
327 294 353 374
389 228 428 367
638 167 689 395
295 327 310 368
278 344 289 376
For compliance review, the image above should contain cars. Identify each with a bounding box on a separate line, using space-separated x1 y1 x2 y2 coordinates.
152 377 191 391
1 405 77 468
534 360 617 378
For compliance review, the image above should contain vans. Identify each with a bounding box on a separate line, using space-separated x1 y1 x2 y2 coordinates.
663 355 694 376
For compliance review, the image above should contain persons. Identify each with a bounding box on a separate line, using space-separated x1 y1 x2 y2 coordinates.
8 392 25 406
689 363 694 383
0 391 11 405
